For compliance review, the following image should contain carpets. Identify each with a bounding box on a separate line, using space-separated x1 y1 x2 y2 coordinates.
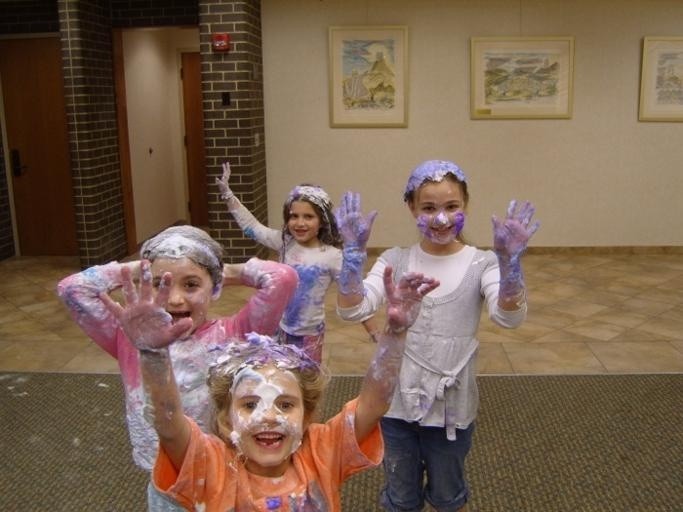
2 371 683 512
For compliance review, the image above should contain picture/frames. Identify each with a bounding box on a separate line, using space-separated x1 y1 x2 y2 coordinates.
328 25 408 128
470 36 575 120
638 35 683 123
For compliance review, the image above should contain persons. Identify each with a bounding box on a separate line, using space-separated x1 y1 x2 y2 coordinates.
332 158 541 512
96 258 442 512
213 159 382 365
55 223 301 511
